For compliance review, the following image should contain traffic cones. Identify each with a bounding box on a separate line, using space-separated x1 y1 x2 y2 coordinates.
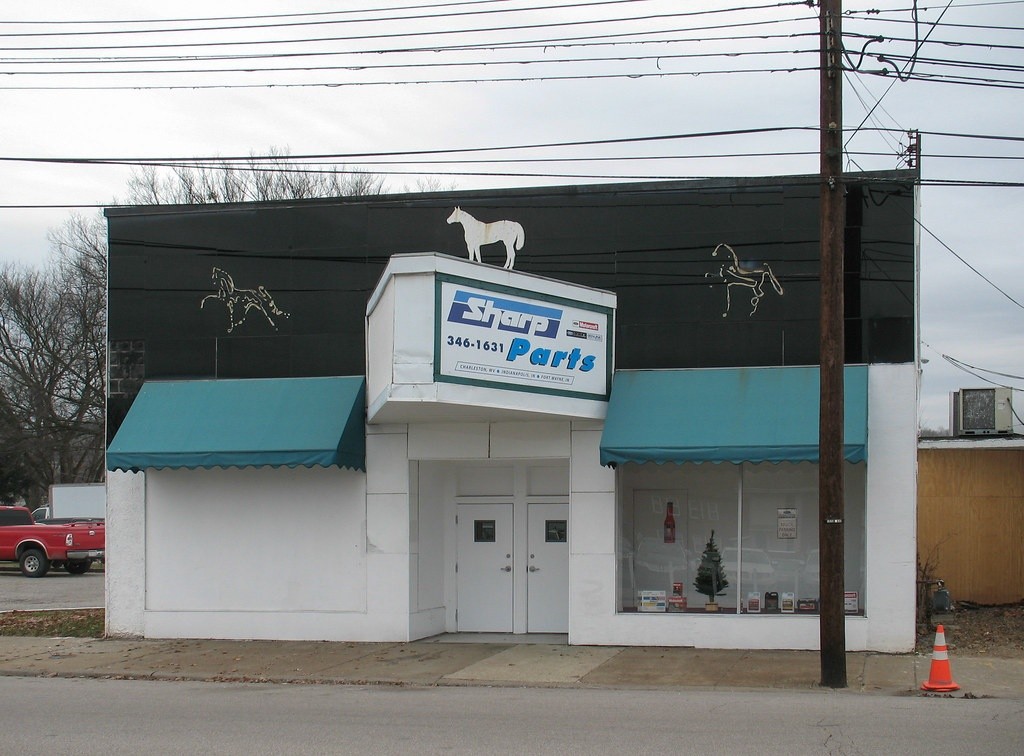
919 623 962 692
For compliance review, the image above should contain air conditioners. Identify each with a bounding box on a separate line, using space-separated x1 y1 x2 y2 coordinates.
948 387 1014 437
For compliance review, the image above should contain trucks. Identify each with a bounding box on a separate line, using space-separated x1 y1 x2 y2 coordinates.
29 483 106 525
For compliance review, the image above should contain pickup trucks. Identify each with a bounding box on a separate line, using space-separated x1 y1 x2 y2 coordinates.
0 506 104 579
